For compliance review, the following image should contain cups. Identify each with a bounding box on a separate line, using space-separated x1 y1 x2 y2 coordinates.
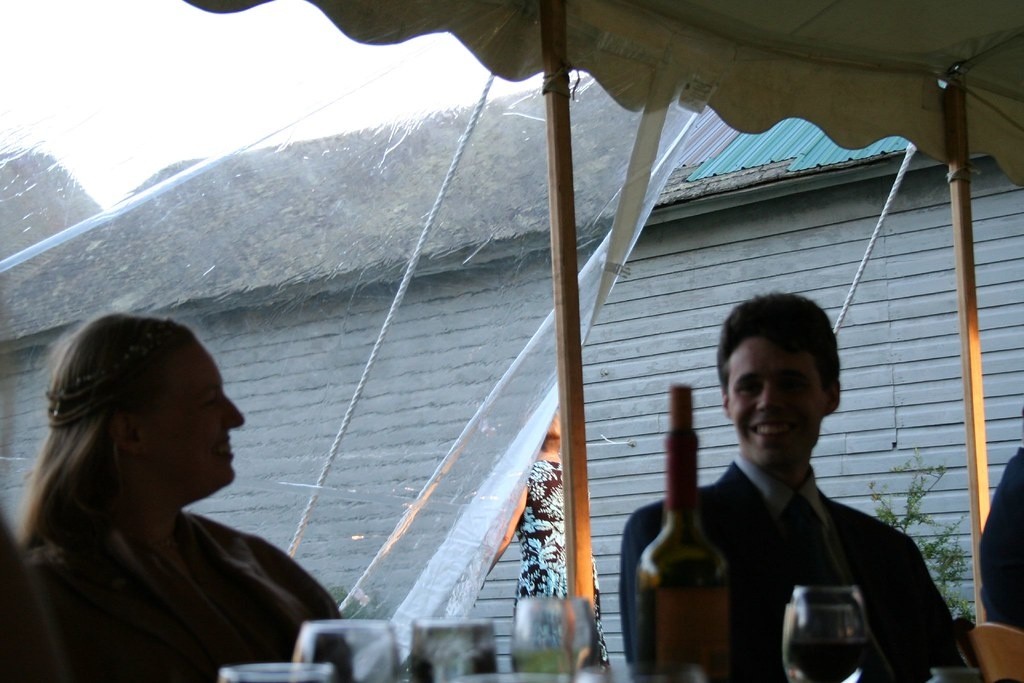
411 617 498 683
786 584 870 683
289 619 399 683
217 661 338 683
781 602 865 683
510 597 600 674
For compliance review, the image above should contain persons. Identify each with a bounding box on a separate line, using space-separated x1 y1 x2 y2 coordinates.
620 292 968 682
474 418 610 662
0 314 354 683
979 447 1024 631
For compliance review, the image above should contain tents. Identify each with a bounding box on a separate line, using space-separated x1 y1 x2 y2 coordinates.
185 0 1023 624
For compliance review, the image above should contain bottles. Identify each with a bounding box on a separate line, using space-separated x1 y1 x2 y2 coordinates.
632 383 734 683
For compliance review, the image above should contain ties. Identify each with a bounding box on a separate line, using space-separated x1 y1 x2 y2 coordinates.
788 500 844 587
